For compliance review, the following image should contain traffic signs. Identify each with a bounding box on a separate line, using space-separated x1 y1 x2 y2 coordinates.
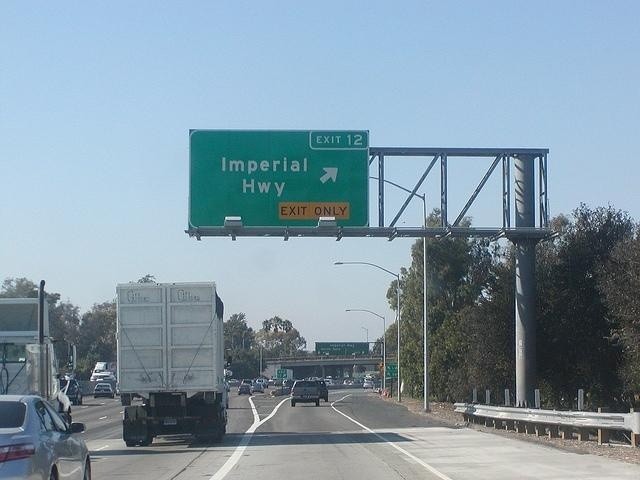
385 364 398 378
277 369 287 378
189 128 370 228
315 342 369 355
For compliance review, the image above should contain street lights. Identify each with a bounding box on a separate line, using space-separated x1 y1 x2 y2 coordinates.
333 261 401 403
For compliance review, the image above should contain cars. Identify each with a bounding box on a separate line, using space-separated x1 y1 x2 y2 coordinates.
0 394 91 480
60 362 116 405
228 373 376 407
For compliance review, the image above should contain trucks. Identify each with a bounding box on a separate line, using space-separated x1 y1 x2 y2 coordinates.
117 282 232 447
0 280 77 426
